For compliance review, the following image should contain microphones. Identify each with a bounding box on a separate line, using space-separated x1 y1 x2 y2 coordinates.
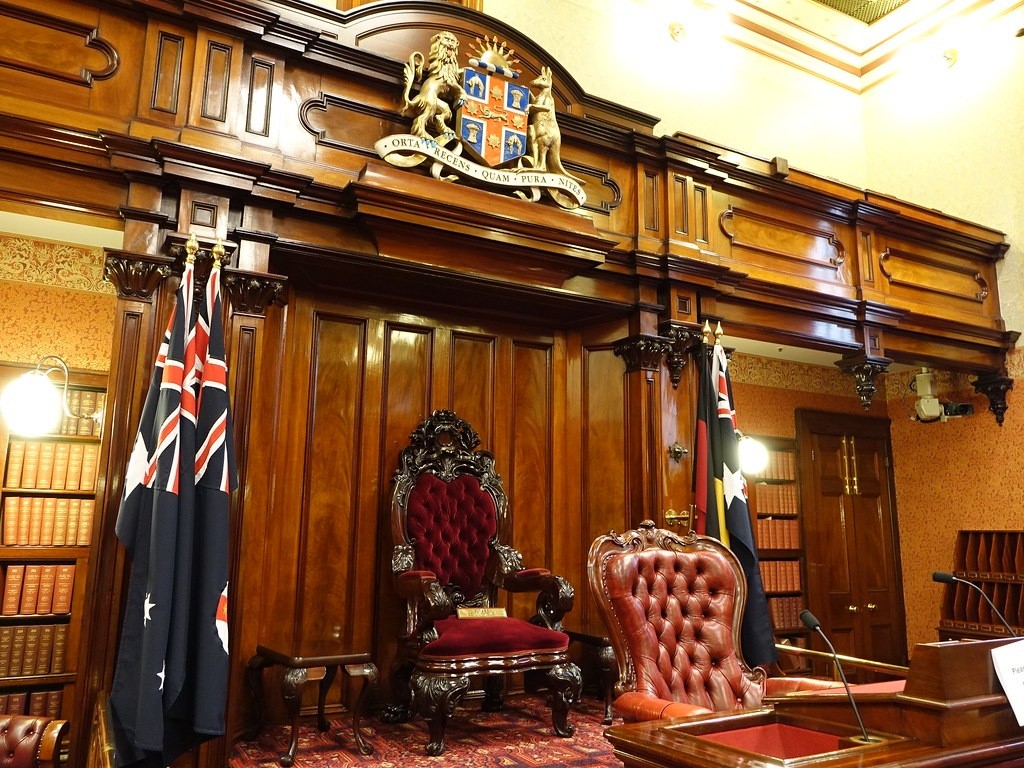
933 572 1017 637
799 609 869 744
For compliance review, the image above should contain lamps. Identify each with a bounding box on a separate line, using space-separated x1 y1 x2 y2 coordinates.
733 429 771 475
0 355 105 440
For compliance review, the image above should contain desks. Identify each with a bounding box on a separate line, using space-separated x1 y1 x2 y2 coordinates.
240 637 378 768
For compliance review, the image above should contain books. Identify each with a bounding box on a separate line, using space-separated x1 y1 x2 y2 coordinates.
0 388 106 719
754 447 807 671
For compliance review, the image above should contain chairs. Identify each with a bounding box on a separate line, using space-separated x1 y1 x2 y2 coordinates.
379 410 585 755
1 714 70 767
587 519 859 734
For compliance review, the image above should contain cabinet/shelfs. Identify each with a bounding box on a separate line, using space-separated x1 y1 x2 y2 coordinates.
0 360 117 767
750 448 810 674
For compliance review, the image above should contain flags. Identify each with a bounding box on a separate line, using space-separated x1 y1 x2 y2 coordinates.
111 261 239 768
691 343 778 670
